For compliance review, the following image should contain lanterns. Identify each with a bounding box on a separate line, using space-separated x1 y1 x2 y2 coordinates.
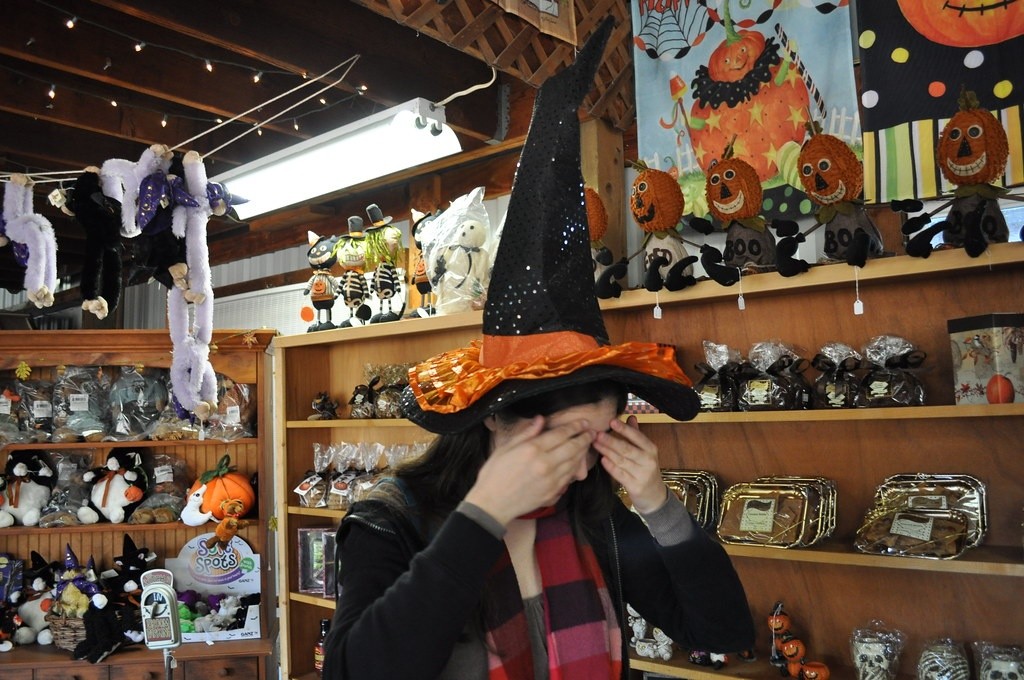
781 636 805 662
768 611 791 634
801 661 829 680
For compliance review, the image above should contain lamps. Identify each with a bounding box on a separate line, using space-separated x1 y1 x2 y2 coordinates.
206 97 462 221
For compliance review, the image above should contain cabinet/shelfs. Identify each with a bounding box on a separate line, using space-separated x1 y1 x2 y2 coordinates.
1 329 276 679
281 241 1022 679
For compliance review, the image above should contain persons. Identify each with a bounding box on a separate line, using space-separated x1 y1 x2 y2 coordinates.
410 208 442 319
330 216 371 329
303 230 341 334
319 327 756 680
363 204 405 324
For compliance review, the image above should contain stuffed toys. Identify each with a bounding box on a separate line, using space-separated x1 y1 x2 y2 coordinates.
51 366 112 443
613 158 697 293
171 179 251 240
204 384 258 440
180 482 222 527
0 173 58 309
100 374 168 443
46 166 123 320
14 378 54 443
204 498 249 550
900 82 1024 260
165 149 220 427
121 152 205 305
99 143 199 239
76 447 153 525
37 451 94 530
127 453 191 526
150 372 226 441
431 219 489 316
698 133 778 287
185 453 255 521
774 104 924 278
581 173 624 299
0 532 261 665
0 448 58 529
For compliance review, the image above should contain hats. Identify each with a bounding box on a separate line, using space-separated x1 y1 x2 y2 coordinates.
398 12 699 436
